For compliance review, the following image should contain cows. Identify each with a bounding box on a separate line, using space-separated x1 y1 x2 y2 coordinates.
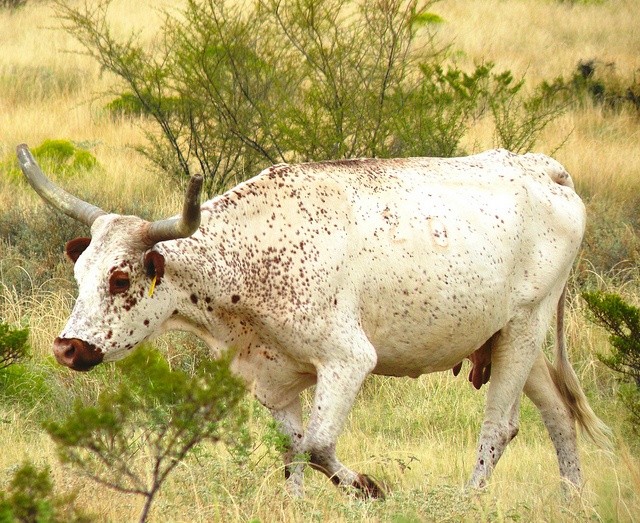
16 142 617 504
